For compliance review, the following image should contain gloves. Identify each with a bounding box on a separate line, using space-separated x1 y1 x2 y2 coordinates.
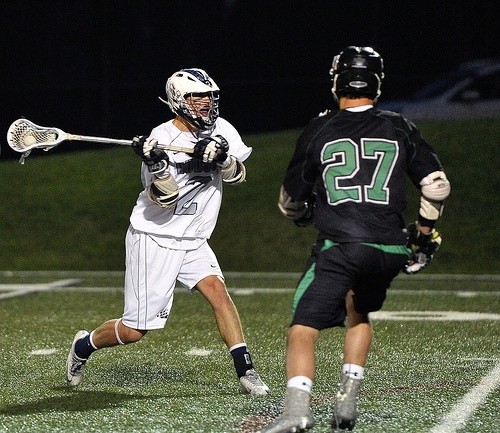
132 135 169 174
185 134 230 165
402 221 443 273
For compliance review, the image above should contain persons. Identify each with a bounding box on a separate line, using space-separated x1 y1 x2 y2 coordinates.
258 47 451 433
65 69 271 396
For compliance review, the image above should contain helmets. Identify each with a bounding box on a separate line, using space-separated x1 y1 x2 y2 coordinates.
166 68 221 131
330 46 384 103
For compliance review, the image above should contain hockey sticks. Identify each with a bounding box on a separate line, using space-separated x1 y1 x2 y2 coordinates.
5 117 190 155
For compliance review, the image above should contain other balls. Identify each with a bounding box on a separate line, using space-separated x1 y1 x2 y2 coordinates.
24 136 36 146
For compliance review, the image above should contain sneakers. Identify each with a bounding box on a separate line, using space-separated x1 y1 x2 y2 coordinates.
66 330 90 387
331 395 358 430
240 368 270 395
259 409 314 433
